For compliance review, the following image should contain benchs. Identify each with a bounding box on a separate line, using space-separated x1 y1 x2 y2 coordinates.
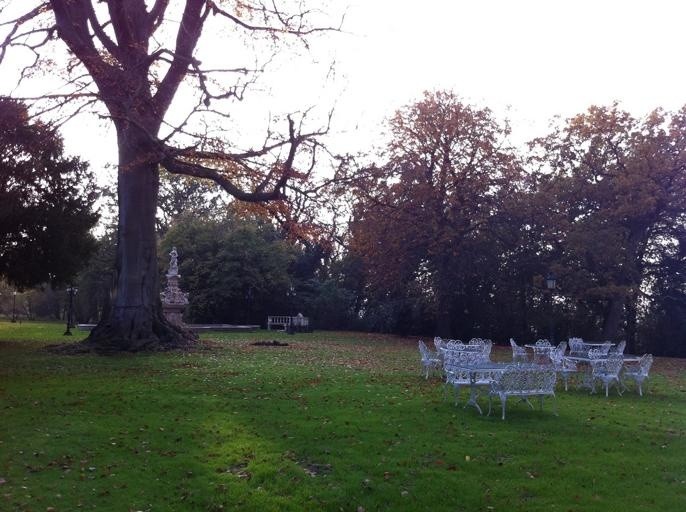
486 363 558 420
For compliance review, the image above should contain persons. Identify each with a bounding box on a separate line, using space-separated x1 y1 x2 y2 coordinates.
168 246 178 274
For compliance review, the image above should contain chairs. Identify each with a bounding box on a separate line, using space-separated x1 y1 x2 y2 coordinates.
417 336 654 398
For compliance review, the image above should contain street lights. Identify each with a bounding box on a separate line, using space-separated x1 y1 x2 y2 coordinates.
546 271 558 362
11 289 17 323
66 286 79 329
285 280 297 315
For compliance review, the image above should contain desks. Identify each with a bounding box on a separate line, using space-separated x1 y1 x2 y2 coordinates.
445 362 578 416
